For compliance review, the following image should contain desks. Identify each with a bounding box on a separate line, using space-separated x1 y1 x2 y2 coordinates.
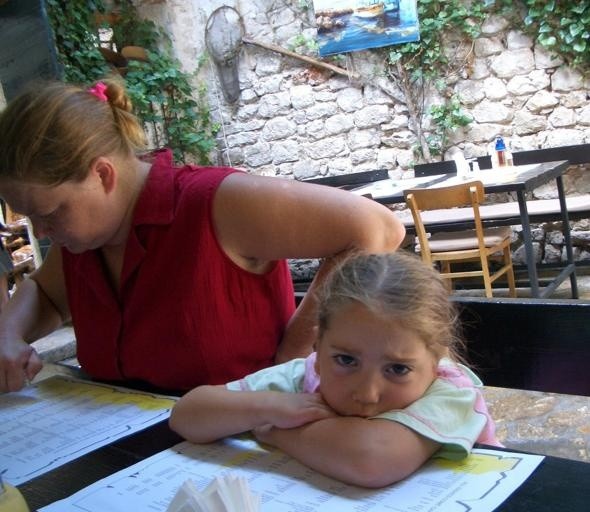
0 372 589 511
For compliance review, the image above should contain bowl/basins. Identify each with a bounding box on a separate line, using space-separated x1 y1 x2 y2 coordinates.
388 169 404 180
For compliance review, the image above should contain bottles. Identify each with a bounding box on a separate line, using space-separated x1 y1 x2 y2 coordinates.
495 136 506 167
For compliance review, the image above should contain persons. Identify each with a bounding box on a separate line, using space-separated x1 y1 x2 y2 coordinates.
0 221 25 307
0 76 408 398
166 248 502 492
